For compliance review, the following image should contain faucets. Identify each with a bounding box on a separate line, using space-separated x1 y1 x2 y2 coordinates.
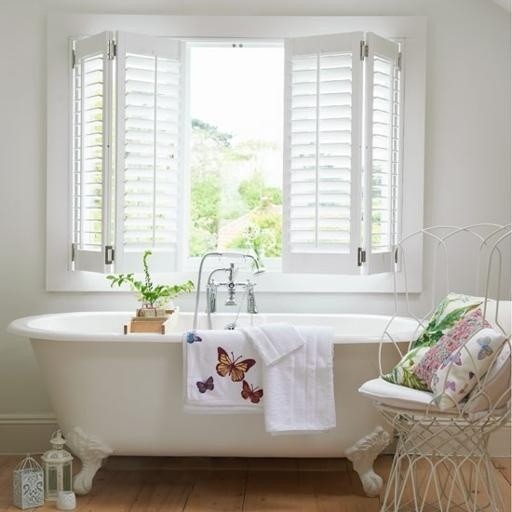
227 261 239 308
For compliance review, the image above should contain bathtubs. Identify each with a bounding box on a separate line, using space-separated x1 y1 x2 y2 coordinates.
7 311 456 458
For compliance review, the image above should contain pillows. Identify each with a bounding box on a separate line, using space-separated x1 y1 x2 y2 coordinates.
383 292 508 412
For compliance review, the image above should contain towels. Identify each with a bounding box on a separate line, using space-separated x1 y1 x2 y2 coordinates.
184 323 337 435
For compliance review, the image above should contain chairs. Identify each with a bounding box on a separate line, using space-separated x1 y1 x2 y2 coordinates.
357 220 511 511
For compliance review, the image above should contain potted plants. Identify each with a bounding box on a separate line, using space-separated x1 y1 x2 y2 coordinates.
106 250 196 318
